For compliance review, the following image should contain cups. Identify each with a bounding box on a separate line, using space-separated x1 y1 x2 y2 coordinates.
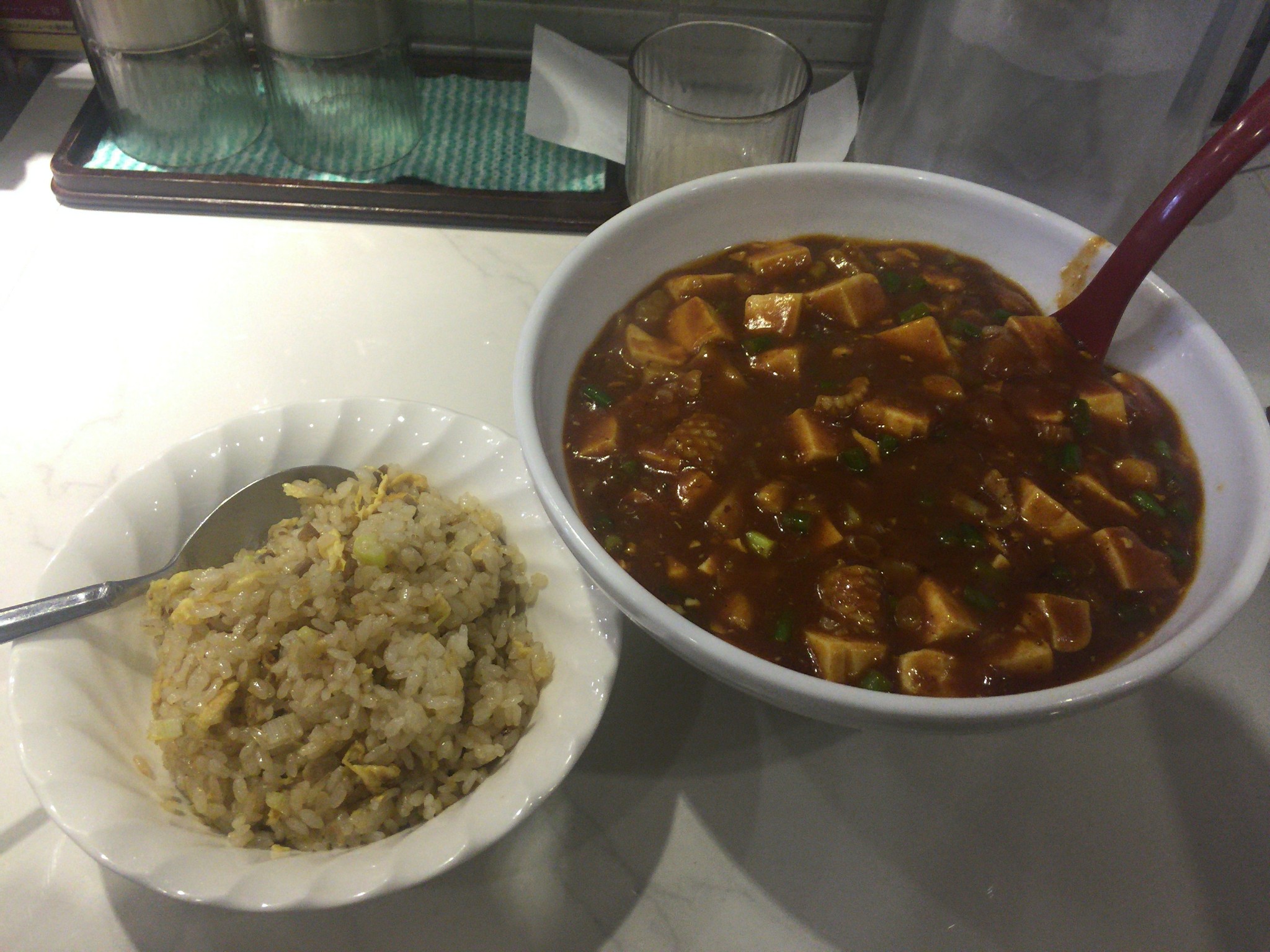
71 0 424 173
624 21 814 206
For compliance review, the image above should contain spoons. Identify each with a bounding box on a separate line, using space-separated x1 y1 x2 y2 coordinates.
0 465 358 645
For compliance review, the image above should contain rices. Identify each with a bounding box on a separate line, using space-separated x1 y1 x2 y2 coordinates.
120 467 558 858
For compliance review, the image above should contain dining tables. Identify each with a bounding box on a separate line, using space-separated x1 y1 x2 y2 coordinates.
2 58 1269 952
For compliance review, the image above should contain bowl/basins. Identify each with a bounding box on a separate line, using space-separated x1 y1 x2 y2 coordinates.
9 396 622 908
510 162 1270 724
